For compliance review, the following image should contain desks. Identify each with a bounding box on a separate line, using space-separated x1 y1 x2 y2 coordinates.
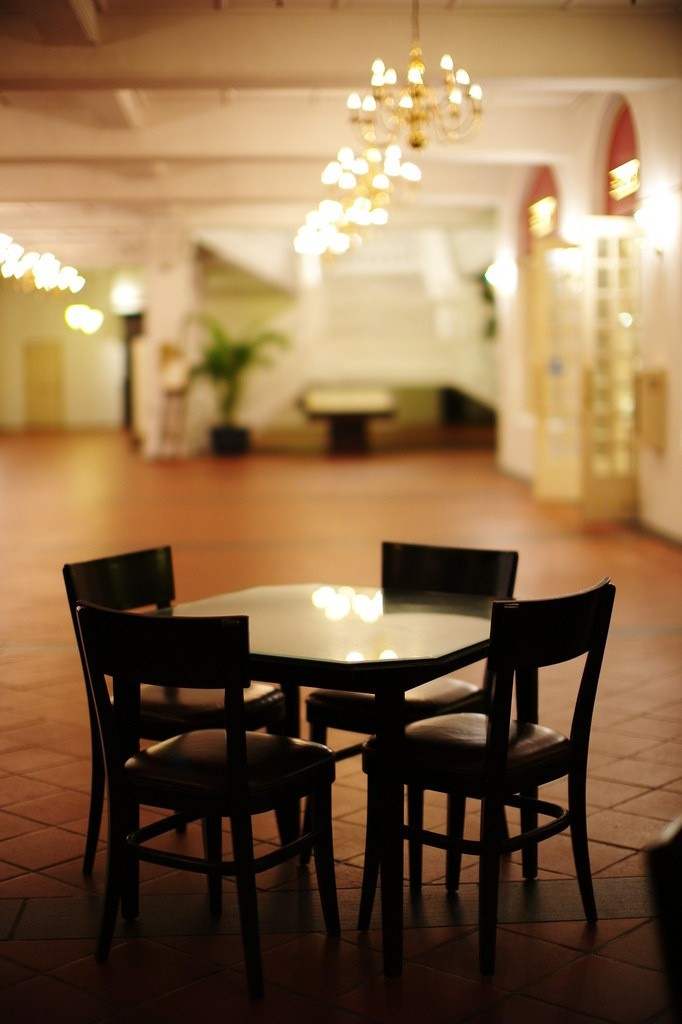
136 580 544 974
303 387 397 458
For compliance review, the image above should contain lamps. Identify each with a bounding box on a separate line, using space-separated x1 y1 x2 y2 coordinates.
344 1 482 156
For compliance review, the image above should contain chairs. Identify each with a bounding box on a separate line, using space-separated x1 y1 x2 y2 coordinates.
298 541 519 868
73 598 341 1001
62 545 298 878
355 574 616 979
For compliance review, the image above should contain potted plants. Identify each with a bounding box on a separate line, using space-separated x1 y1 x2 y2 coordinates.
195 319 294 455
